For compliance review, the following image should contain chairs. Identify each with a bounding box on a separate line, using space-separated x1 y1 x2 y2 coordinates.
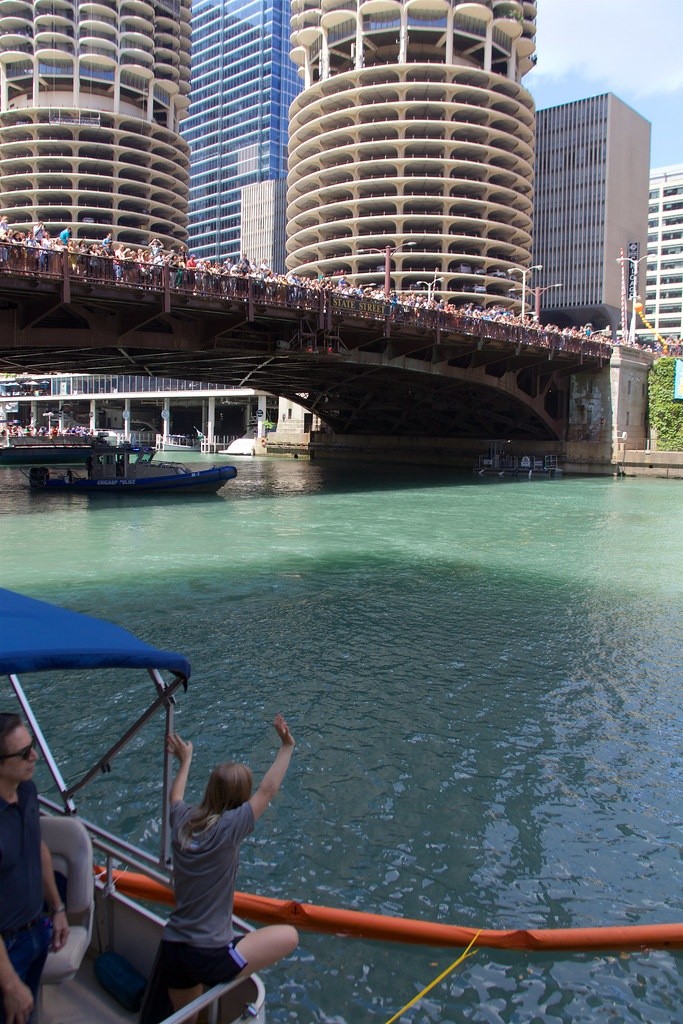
34 816 95 1024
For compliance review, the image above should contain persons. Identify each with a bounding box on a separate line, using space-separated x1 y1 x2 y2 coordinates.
0 215 683 359
148 714 299 1024
0 424 93 437
0 709 89 1024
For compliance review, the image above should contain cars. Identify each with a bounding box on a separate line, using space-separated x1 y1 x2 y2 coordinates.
376 265 385 272
466 284 486 293
452 263 472 274
471 266 486 275
487 269 506 278
82 217 94 223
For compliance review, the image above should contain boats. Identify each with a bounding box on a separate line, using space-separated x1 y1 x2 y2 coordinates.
0 587 268 1024
472 439 563 478
0 434 116 466
44 438 238 495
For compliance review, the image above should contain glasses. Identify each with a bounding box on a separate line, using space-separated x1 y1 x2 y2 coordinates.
0 738 37 762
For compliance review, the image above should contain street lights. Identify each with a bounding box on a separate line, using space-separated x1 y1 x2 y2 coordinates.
616 254 655 318
356 241 417 297
508 284 563 316
417 278 444 302
507 265 543 321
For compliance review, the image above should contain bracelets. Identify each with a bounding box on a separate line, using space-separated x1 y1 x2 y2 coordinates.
48 903 65 916
